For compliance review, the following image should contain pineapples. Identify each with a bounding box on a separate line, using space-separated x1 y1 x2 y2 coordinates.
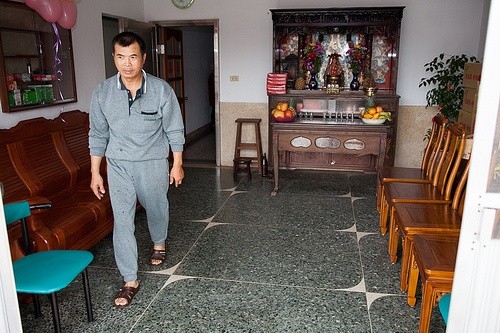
288 99 297 117
294 72 305 90
364 96 376 113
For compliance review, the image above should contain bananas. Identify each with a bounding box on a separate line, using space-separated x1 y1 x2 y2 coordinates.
378 111 391 121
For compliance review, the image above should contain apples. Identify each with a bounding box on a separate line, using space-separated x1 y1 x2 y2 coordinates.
272 101 293 118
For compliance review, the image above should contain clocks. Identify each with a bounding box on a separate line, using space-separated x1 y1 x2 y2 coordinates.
170 0 194 8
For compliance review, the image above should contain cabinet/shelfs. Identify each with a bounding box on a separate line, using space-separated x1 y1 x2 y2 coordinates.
268 6 406 174
0 0 78 112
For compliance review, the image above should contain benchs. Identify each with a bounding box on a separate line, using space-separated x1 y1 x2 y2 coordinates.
0 108 143 305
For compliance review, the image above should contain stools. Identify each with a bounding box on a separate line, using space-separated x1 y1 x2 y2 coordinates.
233 118 264 176
232 157 253 176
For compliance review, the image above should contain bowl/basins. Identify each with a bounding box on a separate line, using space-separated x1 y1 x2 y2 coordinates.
271 113 295 122
361 118 386 125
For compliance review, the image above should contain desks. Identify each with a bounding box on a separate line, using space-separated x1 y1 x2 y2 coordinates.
269 118 391 197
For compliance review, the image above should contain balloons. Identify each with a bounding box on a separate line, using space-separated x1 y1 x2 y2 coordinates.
25 0 62 23
57 0 77 29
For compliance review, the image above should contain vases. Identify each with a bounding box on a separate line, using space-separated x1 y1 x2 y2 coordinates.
350 73 359 91
308 72 318 90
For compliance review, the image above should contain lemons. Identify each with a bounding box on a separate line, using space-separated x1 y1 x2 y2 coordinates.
360 106 382 119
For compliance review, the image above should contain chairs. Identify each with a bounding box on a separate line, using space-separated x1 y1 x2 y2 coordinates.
376 114 472 333
3 200 95 333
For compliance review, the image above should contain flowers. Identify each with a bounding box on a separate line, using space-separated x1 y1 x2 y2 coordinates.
301 40 326 75
345 45 367 75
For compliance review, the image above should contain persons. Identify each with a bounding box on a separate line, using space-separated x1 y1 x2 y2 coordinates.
328 53 342 76
89 32 186 308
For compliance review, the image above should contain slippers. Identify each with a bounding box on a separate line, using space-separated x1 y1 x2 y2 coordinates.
113 283 140 308
149 248 166 266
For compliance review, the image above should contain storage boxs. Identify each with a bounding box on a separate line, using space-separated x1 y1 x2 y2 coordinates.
457 61 482 133
